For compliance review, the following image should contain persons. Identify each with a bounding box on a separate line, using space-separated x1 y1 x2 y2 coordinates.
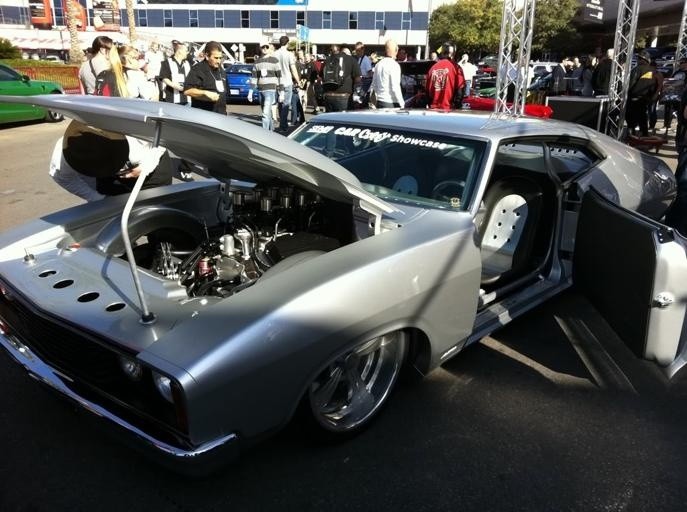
665 88 687 218
277 36 299 126
357 47 375 96
158 40 190 105
663 58 686 142
376 42 406 109
298 50 314 107
429 44 466 109
187 41 228 114
456 54 474 97
552 55 570 99
247 42 284 129
648 61 661 131
592 48 621 134
580 53 597 101
325 44 340 64
145 40 163 77
77 36 124 97
437 42 456 60
49 118 172 202
322 44 360 111
630 53 652 131
117 45 155 98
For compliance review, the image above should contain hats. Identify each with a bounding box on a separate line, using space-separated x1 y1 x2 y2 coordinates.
259 39 271 48
635 49 650 61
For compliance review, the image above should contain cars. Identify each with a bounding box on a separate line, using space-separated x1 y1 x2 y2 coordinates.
223 65 262 103
0 63 66 129
471 80 504 103
532 61 581 83
0 97 687 464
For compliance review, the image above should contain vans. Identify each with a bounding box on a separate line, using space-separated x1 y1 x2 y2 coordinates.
398 61 436 93
